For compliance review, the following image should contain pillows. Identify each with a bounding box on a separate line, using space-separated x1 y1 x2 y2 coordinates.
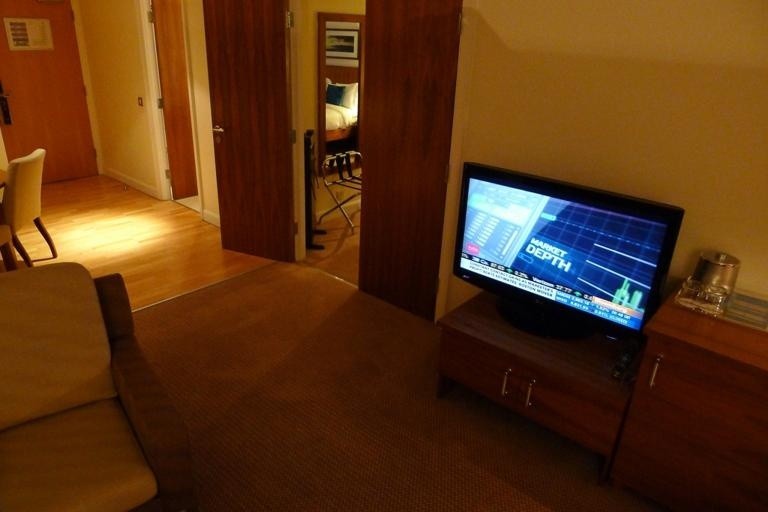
326 82 358 109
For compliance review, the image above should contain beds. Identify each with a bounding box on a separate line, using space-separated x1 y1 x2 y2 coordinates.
325 66 359 142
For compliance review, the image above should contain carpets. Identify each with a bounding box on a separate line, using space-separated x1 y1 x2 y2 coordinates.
133 264 659 509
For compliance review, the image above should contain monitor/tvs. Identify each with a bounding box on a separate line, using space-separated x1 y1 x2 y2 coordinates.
451 160 687 341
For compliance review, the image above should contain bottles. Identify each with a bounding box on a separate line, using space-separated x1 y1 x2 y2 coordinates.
688 249 742 297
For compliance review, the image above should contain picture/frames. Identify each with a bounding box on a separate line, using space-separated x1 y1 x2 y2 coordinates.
326 29 359 59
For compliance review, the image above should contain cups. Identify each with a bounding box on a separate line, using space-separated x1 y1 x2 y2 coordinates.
676 280 728 316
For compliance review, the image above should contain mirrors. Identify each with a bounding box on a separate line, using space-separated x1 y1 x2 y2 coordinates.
318 12 365 177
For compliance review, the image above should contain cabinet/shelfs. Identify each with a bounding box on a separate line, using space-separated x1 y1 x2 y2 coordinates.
436 290 635 482
609 289 767 511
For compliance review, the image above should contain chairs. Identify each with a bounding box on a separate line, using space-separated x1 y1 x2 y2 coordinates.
1 262 201 512
0 148 57 270
